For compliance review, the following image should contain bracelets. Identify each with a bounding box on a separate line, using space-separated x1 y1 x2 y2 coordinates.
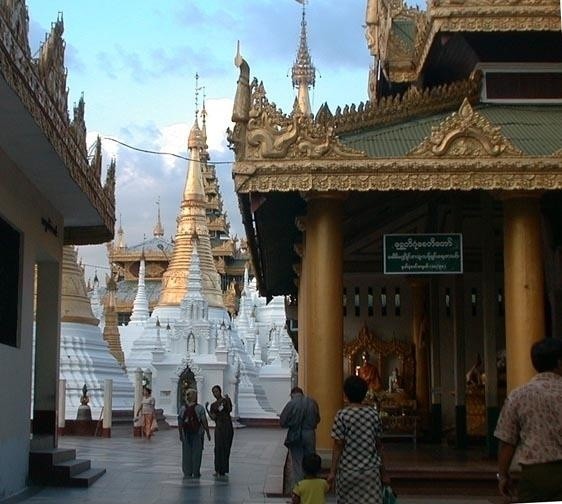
496 472 508 480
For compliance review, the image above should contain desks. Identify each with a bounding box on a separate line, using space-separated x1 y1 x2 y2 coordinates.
371 400 417 443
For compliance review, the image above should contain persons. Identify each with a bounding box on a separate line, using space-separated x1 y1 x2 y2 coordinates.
135 387 157 441
204 385 235 478
290 451 333 503
325 376 392 504
278 386 322 496
356 348 383 392
492 339 561 502
177 388 212 481
387 367 404 393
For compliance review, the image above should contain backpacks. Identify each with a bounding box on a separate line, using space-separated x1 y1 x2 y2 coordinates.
182 404 199 430
